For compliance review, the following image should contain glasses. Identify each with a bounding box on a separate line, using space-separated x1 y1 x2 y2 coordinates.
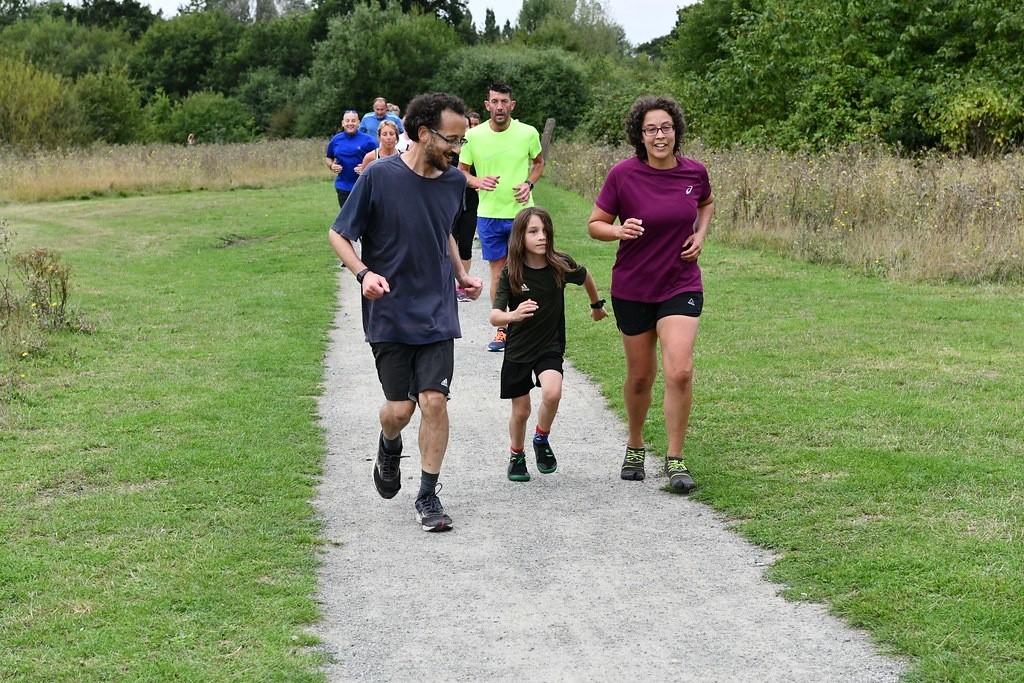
344 110 356 114
430 127 468 147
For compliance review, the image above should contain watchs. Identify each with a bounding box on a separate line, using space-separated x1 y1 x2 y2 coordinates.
356 268 368 284
591 299 606 309
524 181 536 190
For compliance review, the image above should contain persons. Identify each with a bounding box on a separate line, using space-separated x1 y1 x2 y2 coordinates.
457 82 545 352
328 94 483 531
588 96 714 494
326 97 483 301
490 207 609 481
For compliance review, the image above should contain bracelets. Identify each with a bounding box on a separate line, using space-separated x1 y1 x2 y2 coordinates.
329 163 334 171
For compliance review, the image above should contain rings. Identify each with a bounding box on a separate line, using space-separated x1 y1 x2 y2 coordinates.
359 170 361 173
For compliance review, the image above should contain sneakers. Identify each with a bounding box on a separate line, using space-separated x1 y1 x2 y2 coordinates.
507 450 531 481
456 283 473 302
414 483 454 532
533 433 557 474
488 326 508 352
621 445 645 480
373 430 411 499
664 454 697 490
